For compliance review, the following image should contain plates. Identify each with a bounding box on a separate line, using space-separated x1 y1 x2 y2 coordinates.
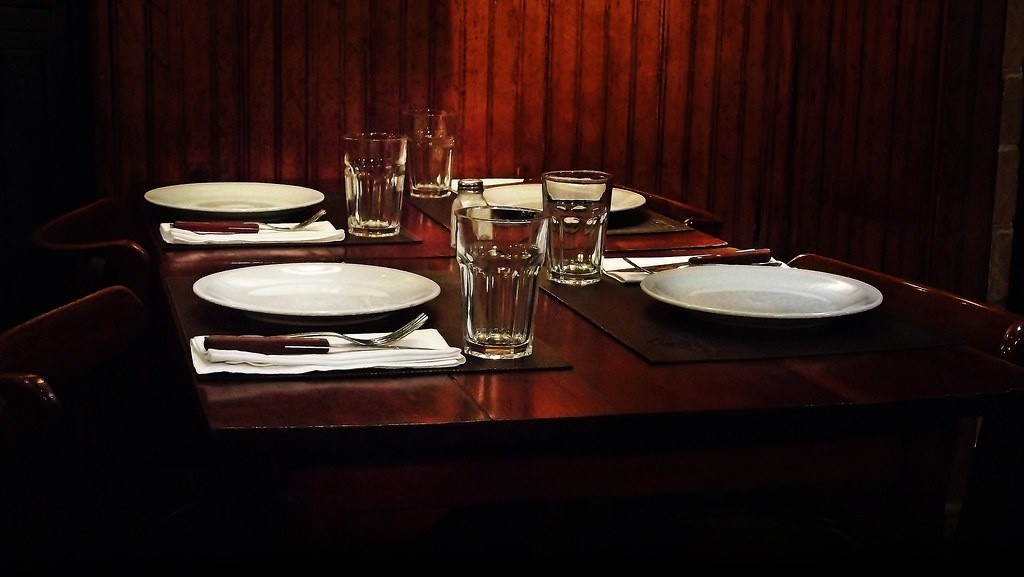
483 183 646 212
641 265 882 318
193 262 441 325
144 182 324 215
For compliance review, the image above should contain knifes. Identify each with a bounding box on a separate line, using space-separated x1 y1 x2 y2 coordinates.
204 336 438 355
607 249 770 273
174 221 319 233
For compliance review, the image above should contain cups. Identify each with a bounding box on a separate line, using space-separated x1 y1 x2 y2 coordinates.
343 132 409 237
543 170 613 286
455 207 548 360
402 109 457 198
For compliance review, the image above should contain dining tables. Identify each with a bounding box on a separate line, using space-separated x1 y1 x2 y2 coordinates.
148 202 1022 472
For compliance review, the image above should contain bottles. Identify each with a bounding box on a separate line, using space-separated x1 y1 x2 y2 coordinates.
450 179 488 249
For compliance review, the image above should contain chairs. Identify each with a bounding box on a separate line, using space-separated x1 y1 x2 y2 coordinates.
614 186 724 237
36 196 154 291
0 286 154 434
786 251 1024 576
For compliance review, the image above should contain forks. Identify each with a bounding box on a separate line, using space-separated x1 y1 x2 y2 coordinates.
245 209 326 231
274 313 428 346
623 256 780 276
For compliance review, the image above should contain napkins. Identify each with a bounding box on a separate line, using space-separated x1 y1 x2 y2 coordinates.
190 327 467 375
603 248 790 283
451 178 522 195
159 219 345 244
